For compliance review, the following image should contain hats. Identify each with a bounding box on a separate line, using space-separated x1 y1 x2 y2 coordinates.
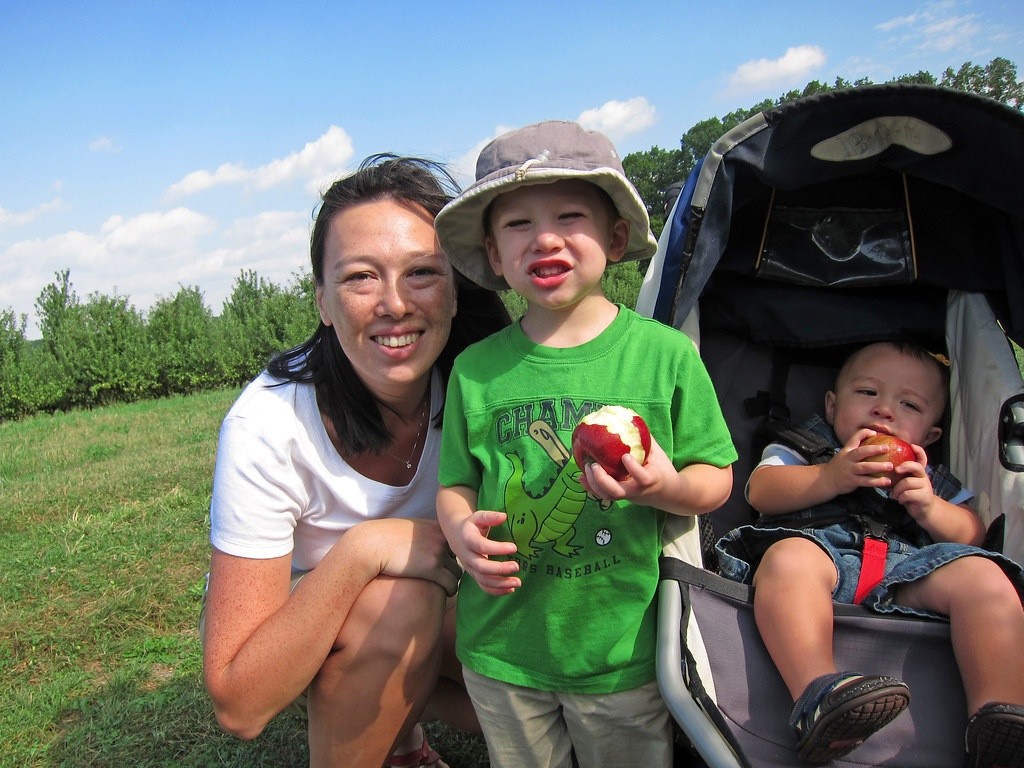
433 120 658 290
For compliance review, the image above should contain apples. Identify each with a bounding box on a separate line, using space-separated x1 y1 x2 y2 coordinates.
571 405 651 481
858 434 917 488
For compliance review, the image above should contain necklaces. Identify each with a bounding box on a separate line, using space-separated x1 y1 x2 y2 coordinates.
385 390 429 470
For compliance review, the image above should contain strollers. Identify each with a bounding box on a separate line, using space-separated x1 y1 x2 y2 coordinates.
616 81 1024 768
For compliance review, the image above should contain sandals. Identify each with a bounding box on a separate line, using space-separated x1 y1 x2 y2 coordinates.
387 728 452 767
789 671 910 764
964 701 1024 768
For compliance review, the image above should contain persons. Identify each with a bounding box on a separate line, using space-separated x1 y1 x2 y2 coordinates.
434 118 740 768
201 157 512 768
742 337 1024 767
